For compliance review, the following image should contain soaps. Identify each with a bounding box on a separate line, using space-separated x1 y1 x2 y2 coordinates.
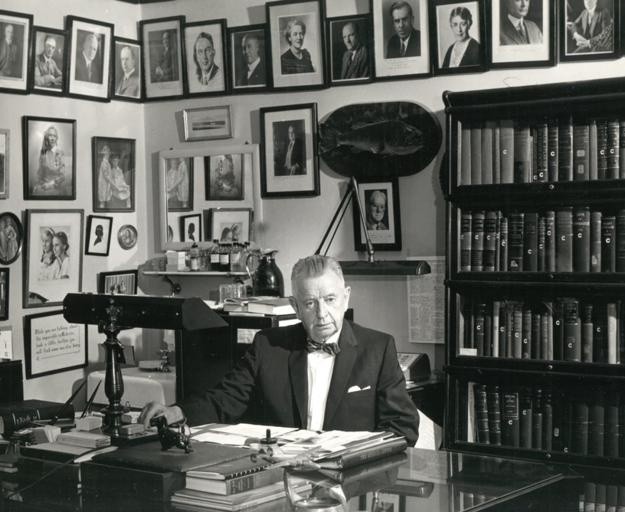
139 360 163 369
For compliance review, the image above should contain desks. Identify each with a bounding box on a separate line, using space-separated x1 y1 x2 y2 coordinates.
0 408 592 510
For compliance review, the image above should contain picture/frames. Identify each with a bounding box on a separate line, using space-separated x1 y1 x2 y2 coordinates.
353 178 402 252
1 104 321 380
1 2 625 104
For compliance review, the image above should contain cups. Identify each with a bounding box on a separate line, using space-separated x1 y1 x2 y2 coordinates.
218 283 246 306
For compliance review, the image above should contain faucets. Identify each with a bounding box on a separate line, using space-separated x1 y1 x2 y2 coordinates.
139 349 171 373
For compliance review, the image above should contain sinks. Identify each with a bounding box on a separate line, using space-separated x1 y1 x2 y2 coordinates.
86 364 176 409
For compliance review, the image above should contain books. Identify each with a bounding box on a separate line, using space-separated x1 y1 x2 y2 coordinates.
171 432 408 512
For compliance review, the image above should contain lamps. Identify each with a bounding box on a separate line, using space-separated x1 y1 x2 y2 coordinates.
62 292 231 443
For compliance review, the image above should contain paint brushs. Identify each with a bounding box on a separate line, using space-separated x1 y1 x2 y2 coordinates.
80 380 102 420
50 380 88 425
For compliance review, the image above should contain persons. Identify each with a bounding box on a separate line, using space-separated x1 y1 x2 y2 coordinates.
98 143 131 208
34 126 67 195
41 227 70 279
278 125 304 175
0 217 18 262
168 157 188 207
138 255 420 447
366 190 387 230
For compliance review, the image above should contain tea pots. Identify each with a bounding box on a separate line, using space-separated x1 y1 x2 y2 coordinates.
245 249 285 301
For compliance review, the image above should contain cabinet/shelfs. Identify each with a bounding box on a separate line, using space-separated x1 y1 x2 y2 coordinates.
431 74 625 512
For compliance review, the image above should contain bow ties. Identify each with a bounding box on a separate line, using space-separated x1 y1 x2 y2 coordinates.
303 337 342 356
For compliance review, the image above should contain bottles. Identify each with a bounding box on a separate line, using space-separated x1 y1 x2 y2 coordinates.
259 428 284 458
190 239 252 273
